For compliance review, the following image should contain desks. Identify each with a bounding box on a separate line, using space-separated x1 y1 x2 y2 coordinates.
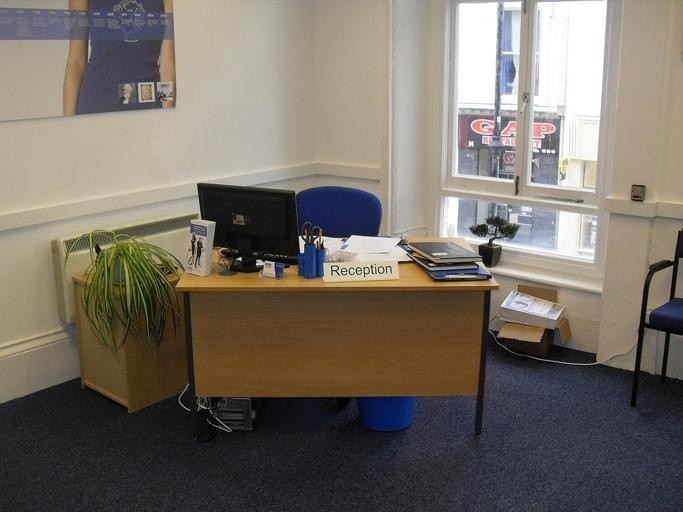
170 239 504 446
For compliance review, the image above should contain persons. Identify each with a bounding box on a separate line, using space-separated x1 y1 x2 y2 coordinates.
61 0 176 116
157 85 168 102
194 237 204 268
119 84 134 104
141 86 151 100
187 231 196 265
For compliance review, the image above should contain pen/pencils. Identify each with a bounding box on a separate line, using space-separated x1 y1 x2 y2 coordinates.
305 227 324 249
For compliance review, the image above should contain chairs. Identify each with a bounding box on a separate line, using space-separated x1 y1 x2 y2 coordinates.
625 226 682 415
290 182 385 246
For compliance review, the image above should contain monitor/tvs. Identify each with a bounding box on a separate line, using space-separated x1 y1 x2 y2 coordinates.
197 183 300 273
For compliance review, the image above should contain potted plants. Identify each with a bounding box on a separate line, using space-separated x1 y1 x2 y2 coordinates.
464 213 523 271
58 225 187 355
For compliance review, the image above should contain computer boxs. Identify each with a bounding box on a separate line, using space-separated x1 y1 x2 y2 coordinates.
214 396 266 430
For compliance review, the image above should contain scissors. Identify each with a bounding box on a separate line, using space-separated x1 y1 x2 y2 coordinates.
301 222 321 244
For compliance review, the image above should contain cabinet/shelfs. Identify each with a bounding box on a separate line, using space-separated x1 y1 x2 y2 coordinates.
64 259 190 416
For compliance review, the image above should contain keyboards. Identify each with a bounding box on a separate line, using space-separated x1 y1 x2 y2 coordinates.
221 248 290 269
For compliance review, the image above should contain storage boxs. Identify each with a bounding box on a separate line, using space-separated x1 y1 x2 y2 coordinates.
485 279 574 368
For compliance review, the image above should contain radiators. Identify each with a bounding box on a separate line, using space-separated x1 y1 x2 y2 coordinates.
54 208 202 332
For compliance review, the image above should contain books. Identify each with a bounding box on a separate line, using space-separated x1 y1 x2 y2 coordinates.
408 241 484 263
423 268 493 282
407 250 480 270
340 235 413 265
497 289 566 330
